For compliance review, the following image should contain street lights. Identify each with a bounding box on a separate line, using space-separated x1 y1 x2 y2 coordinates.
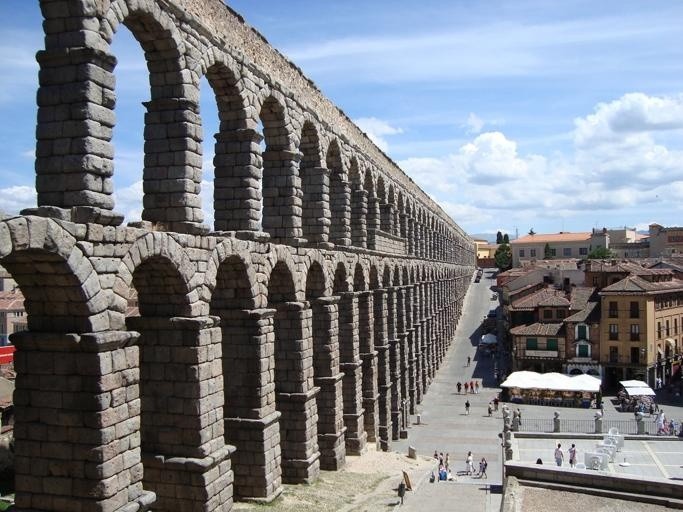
497 432 504 488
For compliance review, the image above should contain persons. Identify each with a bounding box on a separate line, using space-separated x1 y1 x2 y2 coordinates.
466 356 470 366
653 409 680 436
567 443 577 468
657 375 663 391
432 449 488 481
600 401 605 418
455 380 479 394
464 399 471 416
618 388 658 413
486 398 521 425
554 442 563 466
535 456 543 464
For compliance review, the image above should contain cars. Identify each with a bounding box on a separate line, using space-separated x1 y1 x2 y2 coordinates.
473 266 482 283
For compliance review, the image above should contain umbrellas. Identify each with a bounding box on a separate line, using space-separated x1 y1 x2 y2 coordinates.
500 370 655 396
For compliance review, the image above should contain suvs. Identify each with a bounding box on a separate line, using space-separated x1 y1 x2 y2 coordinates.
487 309 495 316
